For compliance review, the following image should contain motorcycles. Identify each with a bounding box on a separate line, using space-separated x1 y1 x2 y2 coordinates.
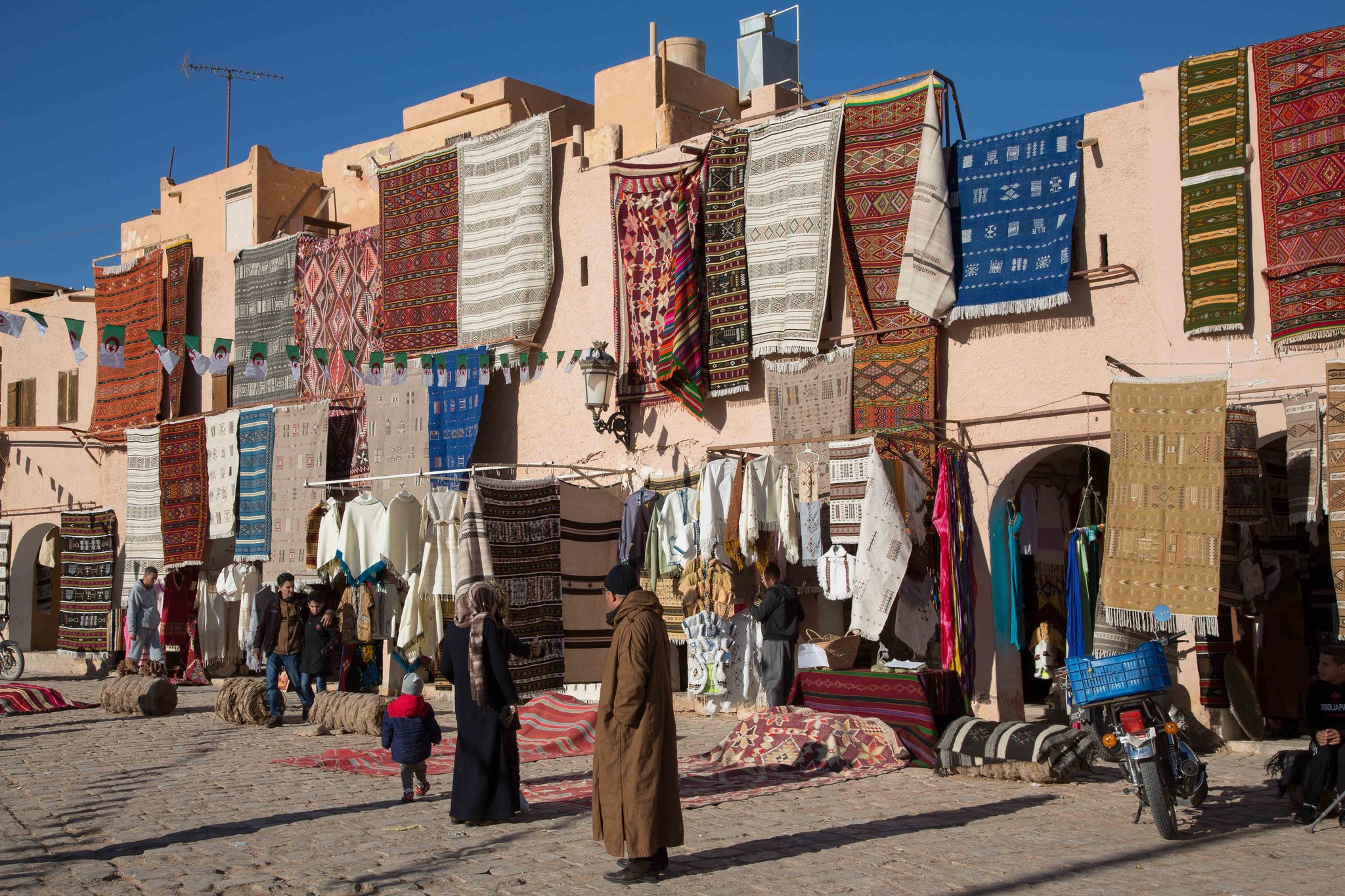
1055 604 1208 840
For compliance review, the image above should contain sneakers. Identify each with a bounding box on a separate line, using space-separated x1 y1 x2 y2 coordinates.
302 707 309 720
264 717 282 727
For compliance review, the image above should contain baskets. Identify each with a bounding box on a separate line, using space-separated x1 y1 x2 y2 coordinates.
806 629 861 670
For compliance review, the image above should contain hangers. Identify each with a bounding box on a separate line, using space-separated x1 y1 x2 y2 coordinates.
795 442 820 478
878 438 905 463
400 483 409 497
701 641 731 659
360 483 373 500
937 430 964 454
742 448 749 472
905 440 923 462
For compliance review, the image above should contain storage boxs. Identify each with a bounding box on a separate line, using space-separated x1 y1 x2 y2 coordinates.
1063 641 1173 705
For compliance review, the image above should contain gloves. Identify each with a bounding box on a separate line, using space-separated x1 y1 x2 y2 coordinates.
748 598 756 608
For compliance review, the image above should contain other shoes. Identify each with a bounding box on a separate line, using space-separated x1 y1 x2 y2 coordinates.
402 791 414 803
465 821 494 827
452 816 463 824
416 781 430 796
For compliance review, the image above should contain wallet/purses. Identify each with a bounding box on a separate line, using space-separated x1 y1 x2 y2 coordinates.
497 705 512 726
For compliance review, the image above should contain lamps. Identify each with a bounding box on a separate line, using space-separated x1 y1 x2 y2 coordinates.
578 340 632 452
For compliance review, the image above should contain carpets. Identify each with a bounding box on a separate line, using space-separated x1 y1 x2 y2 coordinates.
0 24 1345 815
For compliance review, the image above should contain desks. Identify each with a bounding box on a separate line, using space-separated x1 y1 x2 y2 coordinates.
796 670 954 766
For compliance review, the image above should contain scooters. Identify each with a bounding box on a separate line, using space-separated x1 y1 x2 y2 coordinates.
0 614 25 681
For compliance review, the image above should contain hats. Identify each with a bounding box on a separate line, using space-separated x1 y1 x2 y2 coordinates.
401 673 424 696
604 564 639 594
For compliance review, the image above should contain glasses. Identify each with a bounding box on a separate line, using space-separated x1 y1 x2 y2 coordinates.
602 589 615 594
760 575 767 581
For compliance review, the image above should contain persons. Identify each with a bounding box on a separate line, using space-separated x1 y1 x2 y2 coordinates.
440 581 546 827
252 573 335 729
743 561 805 708
1294 639 1345 828
126 566 168 679
299 592 343 708
592 564 684 884
382 672 442 803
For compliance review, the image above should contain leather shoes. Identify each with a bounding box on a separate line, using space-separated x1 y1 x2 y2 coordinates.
604 867 658 884
1339 809 1345 828
1294 805 1316 824
617 858 630 868
